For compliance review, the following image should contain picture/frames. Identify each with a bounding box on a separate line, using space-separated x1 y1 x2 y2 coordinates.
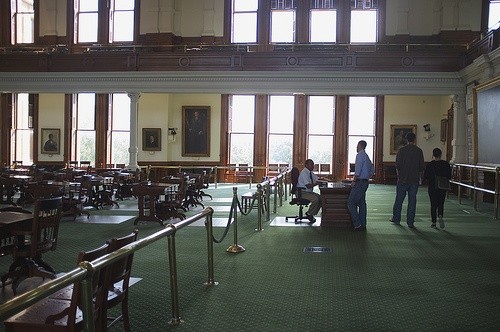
181 105 211 157
389 124 417 156
41 128 61 154
142 127 161 151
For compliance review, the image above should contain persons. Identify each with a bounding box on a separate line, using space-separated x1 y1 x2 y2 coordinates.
295 159 325 223
389 132 424 228
424 148 452 229
347 141 374 232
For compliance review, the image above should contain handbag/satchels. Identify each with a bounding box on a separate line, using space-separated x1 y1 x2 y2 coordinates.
435 176 451 191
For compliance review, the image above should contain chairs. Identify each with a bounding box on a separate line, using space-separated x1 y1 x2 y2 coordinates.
224 164 237 184
320 163 330 179
267 164 278 179
382 166 397 185
349 162 355 177
0 162 218 332
278 163 289 176
236 163 248 184
311 164 320 177
285 167 313 224
241 177 267 215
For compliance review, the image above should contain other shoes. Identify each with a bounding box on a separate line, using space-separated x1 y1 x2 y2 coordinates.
438 215 445 229
408 223 413 227
354 223 364 231
389 217 400 224
362 225 366 231
431 222 436 228
305 212 316 222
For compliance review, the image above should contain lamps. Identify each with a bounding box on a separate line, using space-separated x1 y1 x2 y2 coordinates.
29 115 32 128
424 131 431 140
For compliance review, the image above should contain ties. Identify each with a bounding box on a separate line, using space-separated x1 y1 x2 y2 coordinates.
310 172 313 191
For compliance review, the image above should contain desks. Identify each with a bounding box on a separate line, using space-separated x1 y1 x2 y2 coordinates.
57 168 86 182
318 180 358 228
27 179 82 214
131 181 180 226
100 171 137 200
0 208 56 288
42 172 67 181
74 175 114 210
2 175 37 206
